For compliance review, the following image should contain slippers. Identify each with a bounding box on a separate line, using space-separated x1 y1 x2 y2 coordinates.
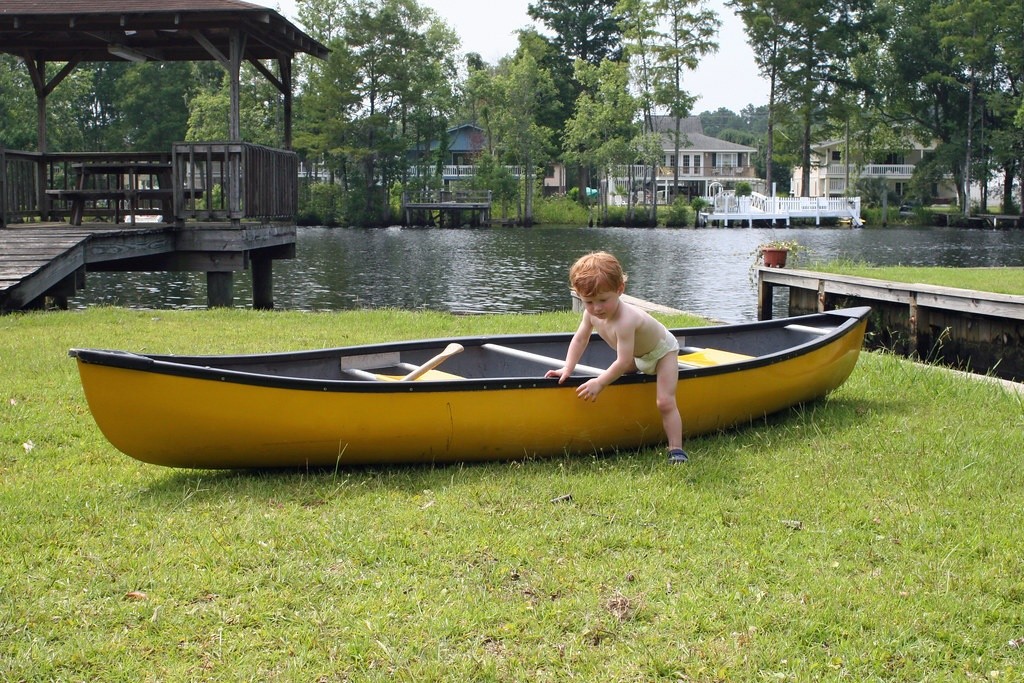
667 449 688 465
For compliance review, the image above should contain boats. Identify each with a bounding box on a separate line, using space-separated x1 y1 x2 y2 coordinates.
67 305 876 470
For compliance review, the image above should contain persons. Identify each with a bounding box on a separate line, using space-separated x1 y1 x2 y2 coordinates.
544 251 689 464
